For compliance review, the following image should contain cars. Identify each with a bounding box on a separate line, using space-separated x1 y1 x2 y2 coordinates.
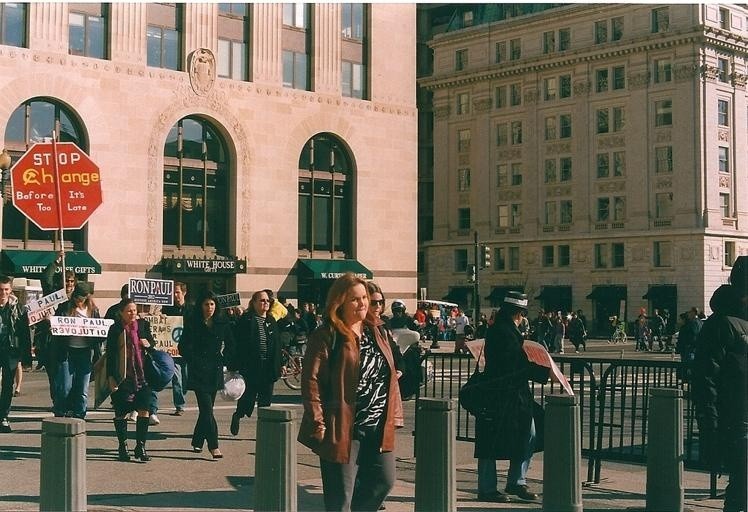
671 316 707 352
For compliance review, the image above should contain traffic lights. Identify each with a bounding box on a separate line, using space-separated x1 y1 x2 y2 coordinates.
486 247 490 268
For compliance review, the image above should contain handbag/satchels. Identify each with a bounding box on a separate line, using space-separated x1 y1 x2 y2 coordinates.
458 342 498 423
221 372 246 402
144 350 176 392
296 331 309 344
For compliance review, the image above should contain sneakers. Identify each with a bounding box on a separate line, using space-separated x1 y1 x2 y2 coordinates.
0 416 11 433
174 409 184 416
14 390 20 397
131 410 139 422
149 414 160 426
230 411 240 436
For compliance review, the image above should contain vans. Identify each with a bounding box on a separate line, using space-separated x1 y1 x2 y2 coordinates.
417 300 459 311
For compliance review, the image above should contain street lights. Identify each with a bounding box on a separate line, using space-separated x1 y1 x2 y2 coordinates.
1 145 42 251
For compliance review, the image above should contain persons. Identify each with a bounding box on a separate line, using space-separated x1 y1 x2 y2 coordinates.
456 291 557 501
2 253 705 472
691 255 747 511
295 274 402 512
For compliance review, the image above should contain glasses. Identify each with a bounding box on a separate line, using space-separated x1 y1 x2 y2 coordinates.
260 299 270 302
370 300 382 306
66 279 74 283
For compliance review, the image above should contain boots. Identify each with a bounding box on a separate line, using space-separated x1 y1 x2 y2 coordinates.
134 415 152 462
112 416 132 461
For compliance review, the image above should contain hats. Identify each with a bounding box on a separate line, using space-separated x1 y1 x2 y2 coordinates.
537 308 544 313
729 254 748 288
641 308 646 313
120 284 128 296
500 290 529 310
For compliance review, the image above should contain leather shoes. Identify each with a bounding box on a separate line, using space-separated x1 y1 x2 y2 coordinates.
191 442 203 453
477 490 511 503
210 447 223 459
504 483 539 501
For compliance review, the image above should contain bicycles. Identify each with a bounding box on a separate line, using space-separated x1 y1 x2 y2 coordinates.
608 326 627 346
275 345 304 393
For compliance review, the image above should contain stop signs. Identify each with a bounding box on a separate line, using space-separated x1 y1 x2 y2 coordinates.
10 142 103 233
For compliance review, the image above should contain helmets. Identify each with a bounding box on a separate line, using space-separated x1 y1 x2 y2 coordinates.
392 300 406 313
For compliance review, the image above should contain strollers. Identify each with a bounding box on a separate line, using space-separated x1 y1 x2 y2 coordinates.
457 324 477 358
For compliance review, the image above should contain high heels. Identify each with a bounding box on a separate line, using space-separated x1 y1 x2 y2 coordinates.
122 412 130 420
